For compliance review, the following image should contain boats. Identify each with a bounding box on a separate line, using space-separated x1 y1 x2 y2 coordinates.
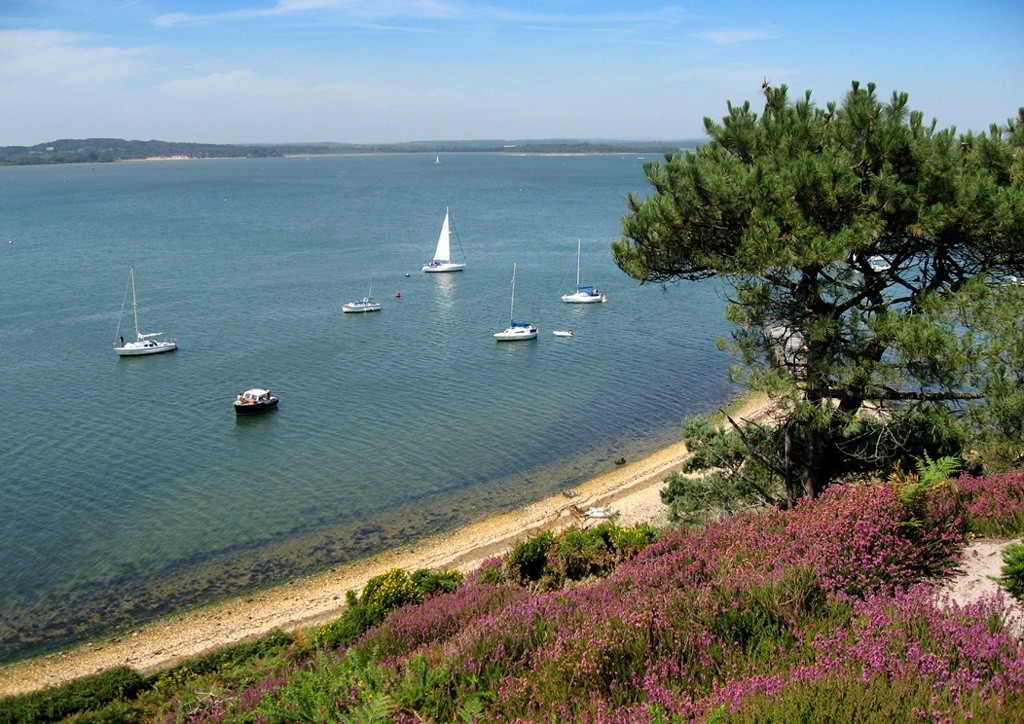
231 389 279 416
342 282 381 313
984 271 1024 288
761 319 810 354
552 330 573 336
845 254 893 273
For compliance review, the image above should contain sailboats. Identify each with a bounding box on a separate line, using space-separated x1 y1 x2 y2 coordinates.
420 207 468 273
492 261 538 341
560 240 608 303
112 266 177 356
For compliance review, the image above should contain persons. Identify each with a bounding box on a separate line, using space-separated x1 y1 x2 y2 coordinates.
236 393 247 402
120 336 124 347
